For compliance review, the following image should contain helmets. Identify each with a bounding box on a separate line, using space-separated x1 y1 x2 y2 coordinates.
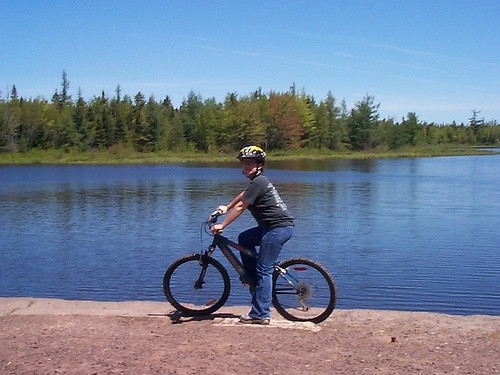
239 146 266 163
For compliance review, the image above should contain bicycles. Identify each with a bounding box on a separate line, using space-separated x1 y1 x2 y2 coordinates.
162 211 337 324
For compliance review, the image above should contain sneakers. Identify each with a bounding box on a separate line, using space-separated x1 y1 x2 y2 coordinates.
240 314 270 325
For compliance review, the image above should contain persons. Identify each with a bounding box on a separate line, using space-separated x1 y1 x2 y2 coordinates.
206 146 295 326
